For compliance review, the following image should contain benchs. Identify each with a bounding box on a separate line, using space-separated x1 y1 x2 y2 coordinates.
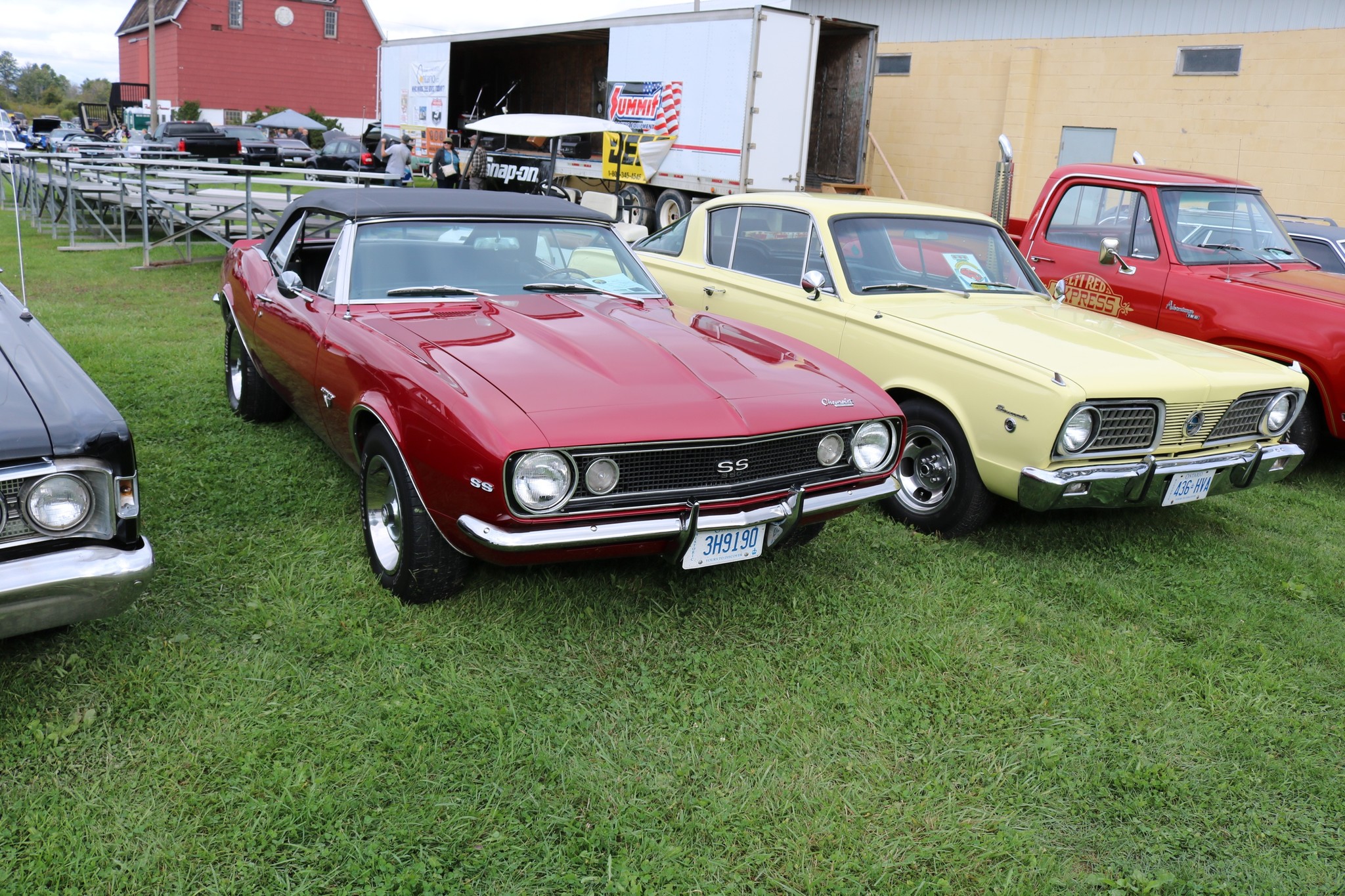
0 142 402 272
543 184 624 220
293 243 541 293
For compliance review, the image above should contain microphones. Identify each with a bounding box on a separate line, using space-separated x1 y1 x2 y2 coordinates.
513 79 521 83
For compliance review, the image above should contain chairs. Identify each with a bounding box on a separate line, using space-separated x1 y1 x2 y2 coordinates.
1134 232 1158 255
1047 232 1092 250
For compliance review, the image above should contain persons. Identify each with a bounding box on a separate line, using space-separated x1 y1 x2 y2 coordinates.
93 121 102 141
432 137 462 189
381 134 412 187
469 135 487 190
274 126 309 146
119 123 131 155
256 125 262 133
141 127 147 142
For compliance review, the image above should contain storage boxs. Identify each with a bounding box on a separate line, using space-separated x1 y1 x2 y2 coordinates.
527 137 546 147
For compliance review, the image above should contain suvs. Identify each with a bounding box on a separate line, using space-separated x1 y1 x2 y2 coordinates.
1097 205 1345 274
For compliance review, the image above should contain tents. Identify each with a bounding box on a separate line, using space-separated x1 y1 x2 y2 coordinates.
255 107 327 131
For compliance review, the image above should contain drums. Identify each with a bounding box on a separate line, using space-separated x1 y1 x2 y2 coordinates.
458 113 481 131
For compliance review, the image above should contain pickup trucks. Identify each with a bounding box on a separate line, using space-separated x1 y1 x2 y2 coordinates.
837 133 1345 469
141 120 243 180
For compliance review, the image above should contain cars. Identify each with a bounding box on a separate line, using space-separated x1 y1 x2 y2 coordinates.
0 108 151 173
568 192 1310 538
213 188 905 608
1 270 154 637
213 122 387 184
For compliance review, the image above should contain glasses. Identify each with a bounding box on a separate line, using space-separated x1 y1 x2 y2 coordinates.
443 141 451 144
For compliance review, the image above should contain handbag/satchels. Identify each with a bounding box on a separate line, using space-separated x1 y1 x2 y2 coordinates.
441 163 456 177
120 137 128 147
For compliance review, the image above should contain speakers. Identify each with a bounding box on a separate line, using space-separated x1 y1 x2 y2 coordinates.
561 136 591 159
373 133 413 163
527 136 548 148
481 137 506 152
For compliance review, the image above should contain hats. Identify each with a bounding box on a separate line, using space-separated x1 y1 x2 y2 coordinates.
257 125 262 129
402 134 411 142
121 123 127 128
279 128 284 133
467 135 478 140
443 137 453 144
